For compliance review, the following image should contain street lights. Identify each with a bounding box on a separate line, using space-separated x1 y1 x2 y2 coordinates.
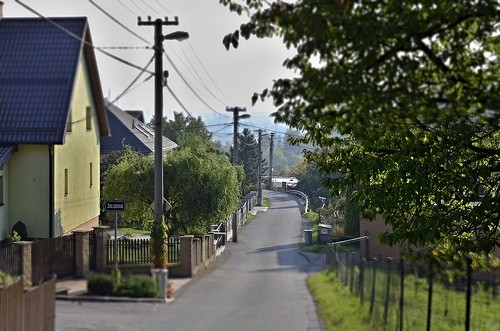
139 13 192 299
226 106 251 243
256 128 270 205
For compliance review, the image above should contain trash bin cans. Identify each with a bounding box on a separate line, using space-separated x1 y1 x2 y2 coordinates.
150 268 168 301
304 229 313 245
